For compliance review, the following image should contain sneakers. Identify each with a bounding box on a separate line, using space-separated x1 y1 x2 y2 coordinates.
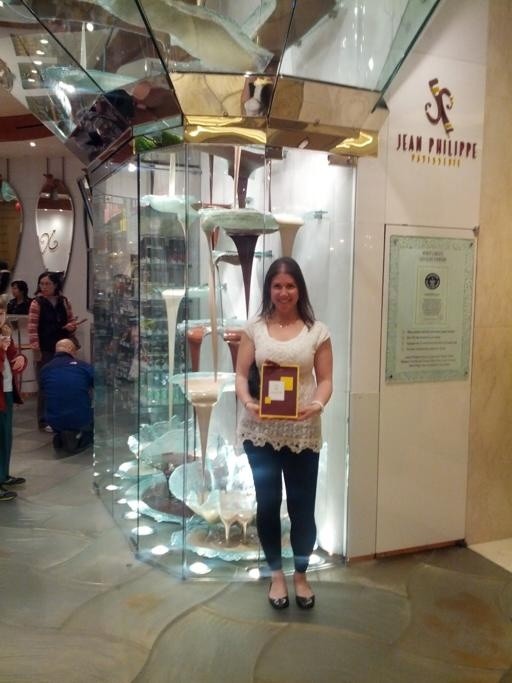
0 485 17 501
0 475 26 486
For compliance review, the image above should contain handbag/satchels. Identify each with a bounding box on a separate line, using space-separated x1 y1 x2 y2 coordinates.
248 359 261 401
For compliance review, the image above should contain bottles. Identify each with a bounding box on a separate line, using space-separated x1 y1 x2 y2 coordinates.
106 231 186 408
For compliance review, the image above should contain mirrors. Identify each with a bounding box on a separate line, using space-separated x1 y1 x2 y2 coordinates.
0 178 24 294
36 177 76 289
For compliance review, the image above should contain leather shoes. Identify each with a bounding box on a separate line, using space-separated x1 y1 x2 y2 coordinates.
268 581 290 610
295 594 316 610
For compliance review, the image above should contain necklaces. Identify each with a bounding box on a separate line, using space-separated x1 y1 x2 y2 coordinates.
270 312 300 328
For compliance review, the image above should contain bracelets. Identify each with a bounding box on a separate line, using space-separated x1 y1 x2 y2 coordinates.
309 401 324 417
244 400 258 408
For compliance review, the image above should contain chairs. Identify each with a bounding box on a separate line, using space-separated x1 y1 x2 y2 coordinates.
8 313 37 393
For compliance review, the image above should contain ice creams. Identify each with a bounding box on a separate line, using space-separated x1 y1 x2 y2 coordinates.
1 325 12 351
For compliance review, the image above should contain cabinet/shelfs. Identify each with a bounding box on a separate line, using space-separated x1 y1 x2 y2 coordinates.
91 234 184 408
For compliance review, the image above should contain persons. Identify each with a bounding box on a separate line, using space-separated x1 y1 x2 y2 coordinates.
39 338 95 454
235 256 332 610
0 293 28 501
7 280 32 315
28 271 77 433
